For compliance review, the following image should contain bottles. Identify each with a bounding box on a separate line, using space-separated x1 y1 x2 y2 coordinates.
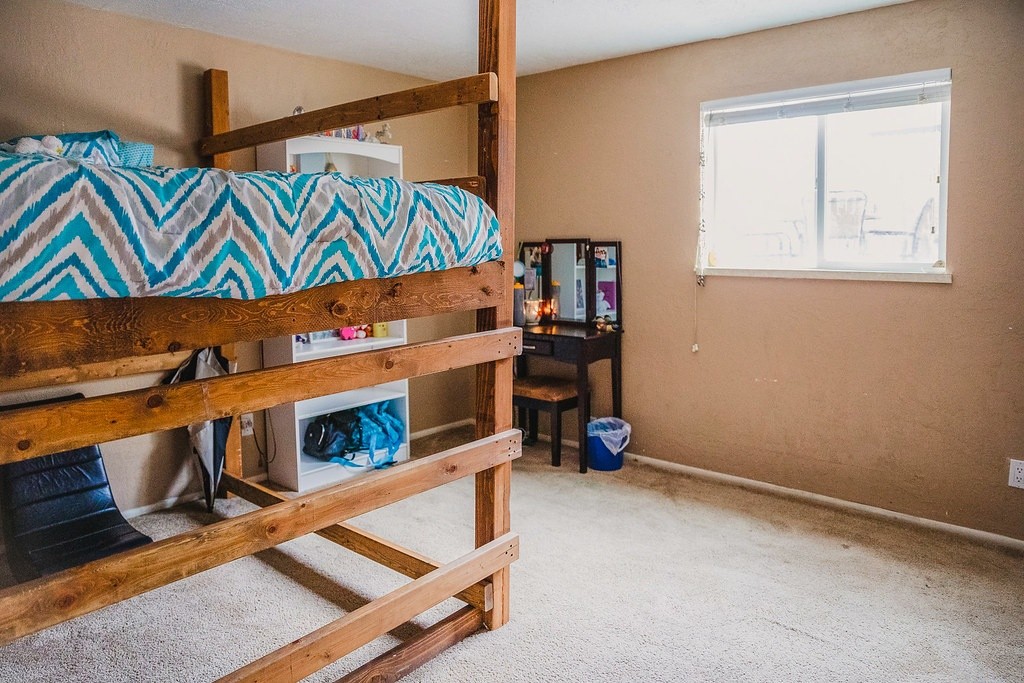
524 300 543 325
373 322 387 337
551 280 561 320
514 282 526 325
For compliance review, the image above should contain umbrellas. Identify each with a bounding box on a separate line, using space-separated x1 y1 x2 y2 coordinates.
168 346 238 513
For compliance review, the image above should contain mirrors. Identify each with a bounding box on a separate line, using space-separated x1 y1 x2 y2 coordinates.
520 236 622 330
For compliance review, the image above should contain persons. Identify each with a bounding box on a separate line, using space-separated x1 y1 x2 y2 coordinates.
595 247 608 268
530 247 540 268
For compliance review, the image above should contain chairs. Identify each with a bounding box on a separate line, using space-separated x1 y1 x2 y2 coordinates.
1 394 155 591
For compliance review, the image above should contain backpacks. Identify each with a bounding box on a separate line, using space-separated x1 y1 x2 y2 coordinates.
302 407 364 461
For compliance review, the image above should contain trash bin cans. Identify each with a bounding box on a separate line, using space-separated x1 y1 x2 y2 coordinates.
587 419 626 471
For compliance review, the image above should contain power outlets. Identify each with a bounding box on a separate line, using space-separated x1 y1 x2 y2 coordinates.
1008 459 1024 489
240 413 254 437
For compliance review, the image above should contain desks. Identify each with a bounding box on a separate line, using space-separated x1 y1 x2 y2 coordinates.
515 322 624 473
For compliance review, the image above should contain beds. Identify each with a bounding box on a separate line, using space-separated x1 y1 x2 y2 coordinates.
1 0 520 682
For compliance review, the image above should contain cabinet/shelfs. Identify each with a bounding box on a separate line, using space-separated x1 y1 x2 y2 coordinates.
260 319 413 493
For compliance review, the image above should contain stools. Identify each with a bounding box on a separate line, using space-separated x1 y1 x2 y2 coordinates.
511 374 591 467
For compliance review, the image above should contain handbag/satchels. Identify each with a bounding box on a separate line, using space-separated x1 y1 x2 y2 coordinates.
354 401 404 468
